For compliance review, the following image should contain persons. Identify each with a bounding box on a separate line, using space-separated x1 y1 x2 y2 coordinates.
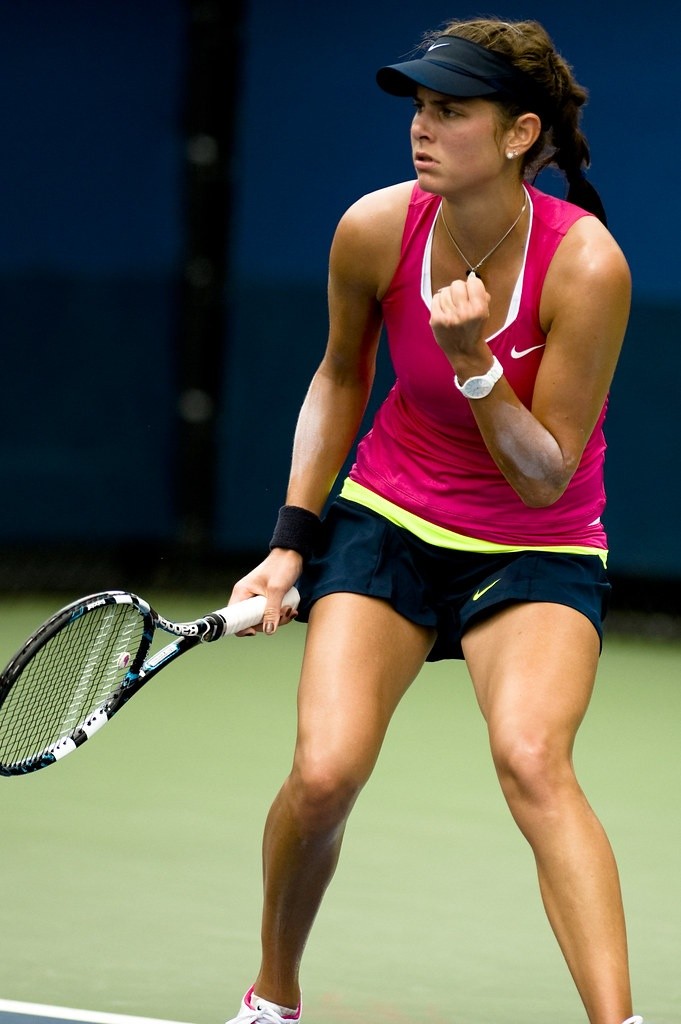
233 20 645 1024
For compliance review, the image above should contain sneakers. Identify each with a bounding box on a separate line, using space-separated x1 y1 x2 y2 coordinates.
225 982 302 1024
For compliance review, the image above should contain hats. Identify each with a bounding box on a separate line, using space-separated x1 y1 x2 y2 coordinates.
375 35 517 98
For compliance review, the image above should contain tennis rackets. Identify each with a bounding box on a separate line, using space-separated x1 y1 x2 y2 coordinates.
0 583 306 778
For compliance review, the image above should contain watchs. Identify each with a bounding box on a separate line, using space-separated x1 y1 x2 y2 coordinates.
454 354 503 400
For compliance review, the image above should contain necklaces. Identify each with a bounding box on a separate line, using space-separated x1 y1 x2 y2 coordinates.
441 193 528 279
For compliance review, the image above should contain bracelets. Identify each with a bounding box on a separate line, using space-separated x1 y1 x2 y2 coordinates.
269 505 322 569
